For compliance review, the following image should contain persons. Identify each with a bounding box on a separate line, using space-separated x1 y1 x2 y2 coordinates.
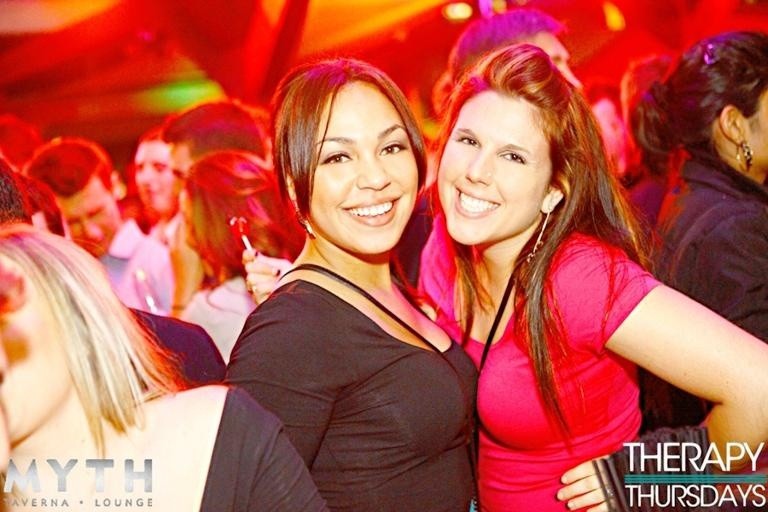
0 11 768 511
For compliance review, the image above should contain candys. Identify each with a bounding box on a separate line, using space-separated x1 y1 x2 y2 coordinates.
230 217 254 258
